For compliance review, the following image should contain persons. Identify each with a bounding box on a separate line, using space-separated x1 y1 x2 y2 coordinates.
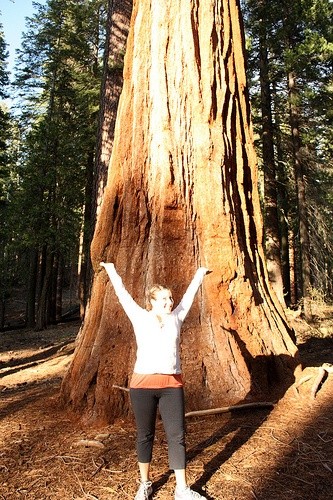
100 262 210 500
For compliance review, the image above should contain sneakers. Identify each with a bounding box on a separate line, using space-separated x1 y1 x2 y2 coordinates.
134 479 153 500
175 486 207 500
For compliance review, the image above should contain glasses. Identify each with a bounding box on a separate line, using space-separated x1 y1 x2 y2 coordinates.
162 297 173 301
